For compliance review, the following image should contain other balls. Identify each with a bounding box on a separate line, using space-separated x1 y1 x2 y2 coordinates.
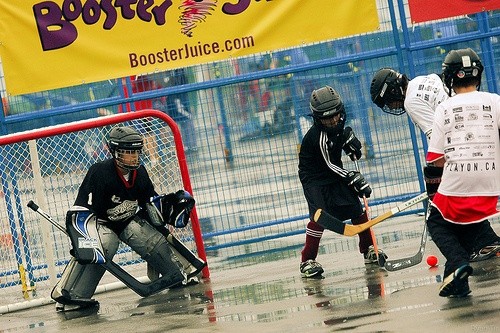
426 255 438 267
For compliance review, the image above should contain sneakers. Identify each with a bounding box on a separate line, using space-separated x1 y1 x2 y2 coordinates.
469 237 500 261
438 262 474 299
300 259 324 277
55 293 99 311
364 244 388 263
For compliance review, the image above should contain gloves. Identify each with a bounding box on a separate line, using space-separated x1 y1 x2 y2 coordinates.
346 171 372 199
339 126 363 162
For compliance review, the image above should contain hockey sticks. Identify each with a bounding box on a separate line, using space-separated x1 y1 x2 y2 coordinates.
26 199 186 299
352 152 387 267
313 190 428 237
384 197 433 273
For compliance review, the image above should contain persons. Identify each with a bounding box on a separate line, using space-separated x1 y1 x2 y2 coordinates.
50 127 195 312
116 77 167 177
423 48 500 297
296 85 389 279
162 71 197 152
370 68 500 261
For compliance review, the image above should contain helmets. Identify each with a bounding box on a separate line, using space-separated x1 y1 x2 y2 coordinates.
310 86 347 134
442 48 485 97
370 69 410 115
106 126 145 171
